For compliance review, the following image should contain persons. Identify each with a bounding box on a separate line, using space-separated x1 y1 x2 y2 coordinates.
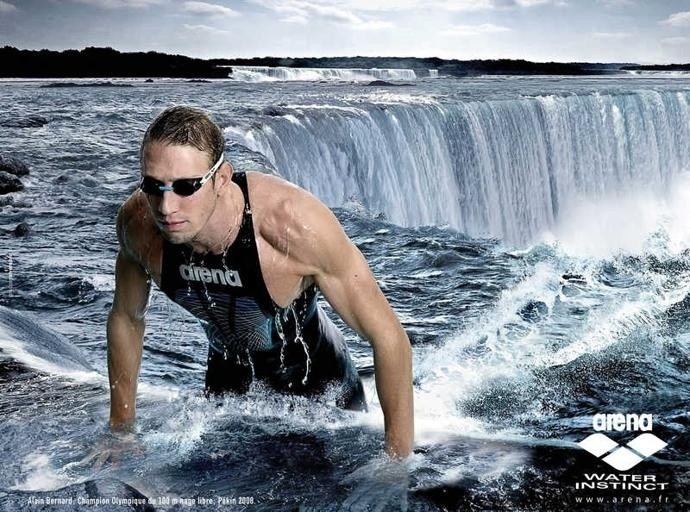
79 105 414 512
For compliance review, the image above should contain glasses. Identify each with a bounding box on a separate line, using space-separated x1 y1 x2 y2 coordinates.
140 154 223 195
